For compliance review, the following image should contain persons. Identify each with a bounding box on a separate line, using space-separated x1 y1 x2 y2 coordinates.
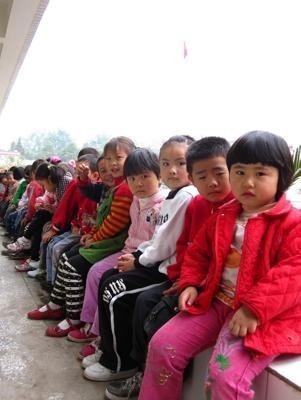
136 129 301 399
1 137 236 399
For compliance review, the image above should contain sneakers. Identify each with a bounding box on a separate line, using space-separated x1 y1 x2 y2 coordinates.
0 222 142 400
